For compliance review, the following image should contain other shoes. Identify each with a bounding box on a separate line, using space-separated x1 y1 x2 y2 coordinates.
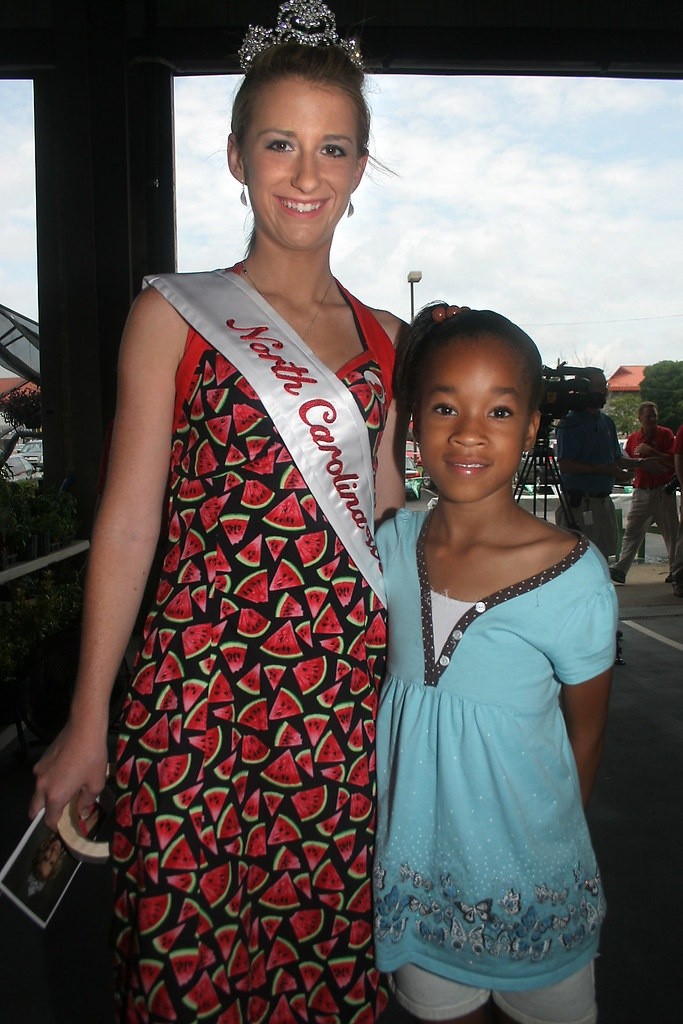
672 579 683 596
609 567 626 583
665 572 672 583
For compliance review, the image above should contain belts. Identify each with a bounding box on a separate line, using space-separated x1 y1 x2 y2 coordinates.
589 492 608 498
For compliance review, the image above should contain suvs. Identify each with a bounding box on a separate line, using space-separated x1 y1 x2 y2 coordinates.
20 440 44 468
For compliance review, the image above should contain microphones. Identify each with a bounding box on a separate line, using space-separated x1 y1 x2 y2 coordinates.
547 366 592 378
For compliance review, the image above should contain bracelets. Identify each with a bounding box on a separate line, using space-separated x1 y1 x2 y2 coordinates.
638 458 645 463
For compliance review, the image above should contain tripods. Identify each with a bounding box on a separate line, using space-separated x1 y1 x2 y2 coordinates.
513 413 580 532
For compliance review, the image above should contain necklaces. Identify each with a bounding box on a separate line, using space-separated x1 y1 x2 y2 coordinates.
243 258 332 341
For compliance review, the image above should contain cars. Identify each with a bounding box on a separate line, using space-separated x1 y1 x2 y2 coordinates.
404 456 422 498
2 456 35 481
17 444 25 452
406 440 422 466
515 438 560 485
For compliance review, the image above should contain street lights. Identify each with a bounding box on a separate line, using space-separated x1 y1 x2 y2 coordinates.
407 269 422 320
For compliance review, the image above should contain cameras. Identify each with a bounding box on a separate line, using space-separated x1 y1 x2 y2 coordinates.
662 473 682 496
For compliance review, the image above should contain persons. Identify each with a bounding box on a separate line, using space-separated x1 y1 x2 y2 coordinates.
670 424 683 597
32 798 98 882
371 302 616 1024
608 402 680 583
30 0 411 1024
554 368 669 664
